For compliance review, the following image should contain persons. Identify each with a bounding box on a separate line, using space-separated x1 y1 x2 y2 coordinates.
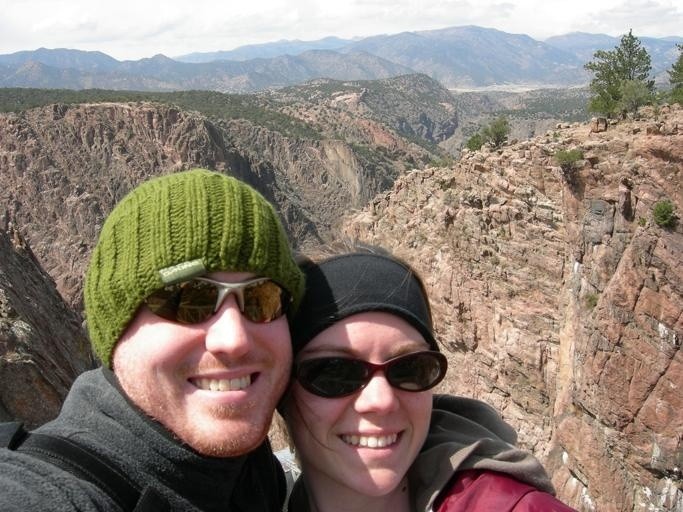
1 167 308 511
272 249 576 512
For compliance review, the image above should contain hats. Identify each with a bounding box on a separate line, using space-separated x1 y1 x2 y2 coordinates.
84 170 305 371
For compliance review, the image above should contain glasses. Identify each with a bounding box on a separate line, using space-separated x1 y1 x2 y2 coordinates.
144 277 293 325
297 350 447 399
